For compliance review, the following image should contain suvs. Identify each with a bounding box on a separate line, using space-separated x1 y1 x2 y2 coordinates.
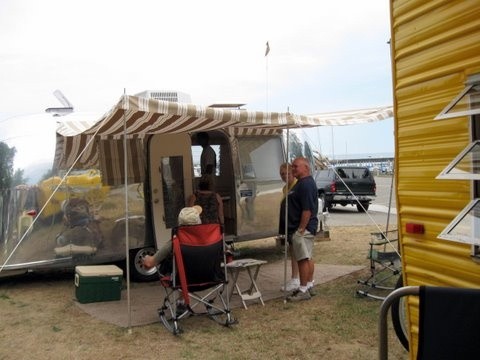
314 165 378 213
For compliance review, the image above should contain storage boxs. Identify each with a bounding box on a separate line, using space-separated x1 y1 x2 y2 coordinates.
74 265 123 304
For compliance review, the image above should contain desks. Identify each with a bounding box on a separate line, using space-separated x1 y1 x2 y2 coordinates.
225 258 268 310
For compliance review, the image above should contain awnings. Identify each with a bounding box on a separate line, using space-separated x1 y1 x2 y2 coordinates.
53 89 395 324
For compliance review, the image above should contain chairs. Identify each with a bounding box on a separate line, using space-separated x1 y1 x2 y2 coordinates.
355 229 404 300
156 222 239 337
378 285 480 360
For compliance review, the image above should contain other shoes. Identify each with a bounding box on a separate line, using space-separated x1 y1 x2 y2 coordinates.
287 289 311 302
281 278 301 292
176 304 186 314
308 287 316 296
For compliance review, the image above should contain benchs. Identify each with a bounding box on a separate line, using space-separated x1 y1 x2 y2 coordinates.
196 191 232 220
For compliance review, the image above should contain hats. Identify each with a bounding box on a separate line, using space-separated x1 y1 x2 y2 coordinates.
178 205 202 225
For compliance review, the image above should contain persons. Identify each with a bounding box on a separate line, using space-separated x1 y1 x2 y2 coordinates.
194 131 217 191
188 174 224 224
278 156 319 300
280 162 301 291
139 206 233 317
